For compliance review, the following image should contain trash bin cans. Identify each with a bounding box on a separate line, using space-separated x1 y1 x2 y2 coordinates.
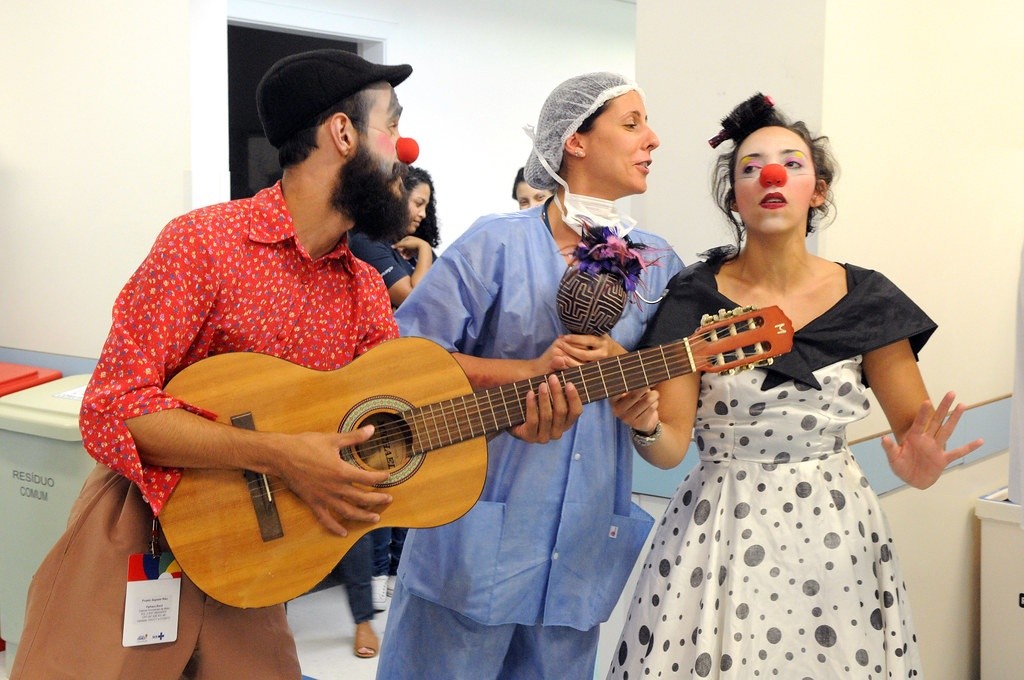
0 363 107 680
975 486 1024 680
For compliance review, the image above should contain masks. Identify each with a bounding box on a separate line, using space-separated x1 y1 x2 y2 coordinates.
562 193 640 241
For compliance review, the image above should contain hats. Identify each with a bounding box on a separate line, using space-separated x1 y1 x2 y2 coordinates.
256 49 412 150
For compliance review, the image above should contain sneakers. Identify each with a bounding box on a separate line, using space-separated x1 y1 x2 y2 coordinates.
387 575 398 596
371 574 389 611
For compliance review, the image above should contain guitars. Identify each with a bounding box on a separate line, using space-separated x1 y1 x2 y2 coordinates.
158 306 796 609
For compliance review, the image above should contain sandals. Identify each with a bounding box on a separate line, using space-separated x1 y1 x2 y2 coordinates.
355 622 379 658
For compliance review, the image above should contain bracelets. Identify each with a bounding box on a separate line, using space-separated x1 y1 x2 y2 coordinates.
630 420 662 447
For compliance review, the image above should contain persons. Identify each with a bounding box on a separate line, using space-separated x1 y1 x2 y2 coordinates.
373 72 686 680
512 167 555 211
284 165 440 658
607 92 986 680
6 48 583 680
